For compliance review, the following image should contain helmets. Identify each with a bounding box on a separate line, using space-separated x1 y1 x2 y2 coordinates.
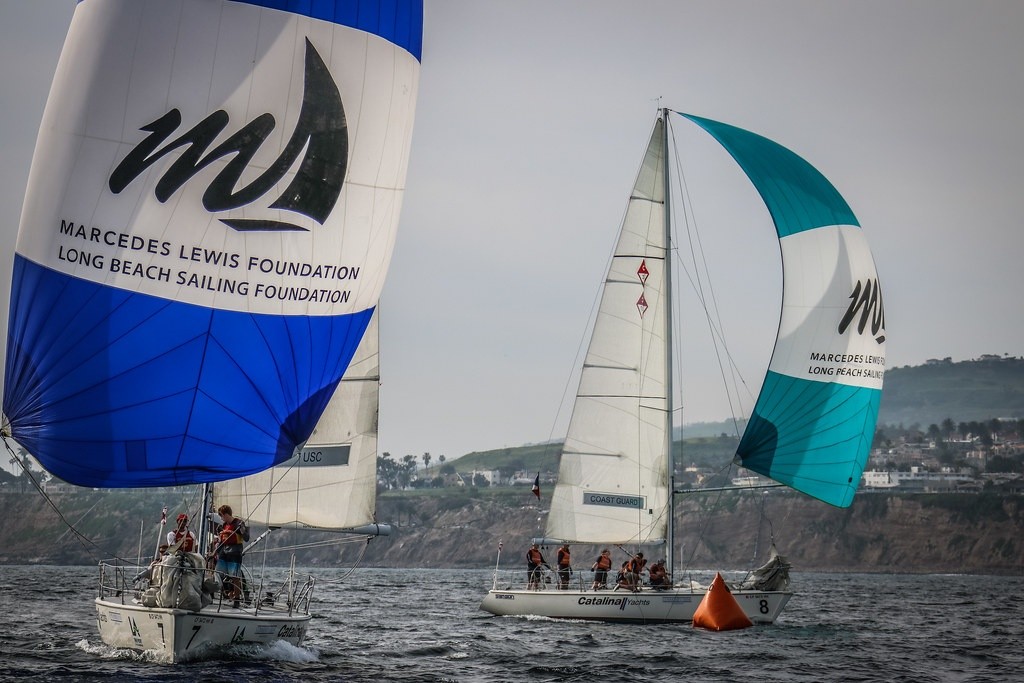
658 558 665 564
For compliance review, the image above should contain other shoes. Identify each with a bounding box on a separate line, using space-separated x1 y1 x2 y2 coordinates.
232 598 240 609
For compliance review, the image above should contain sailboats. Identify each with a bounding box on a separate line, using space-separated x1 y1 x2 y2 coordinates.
1 0 425 666
477 85 888 626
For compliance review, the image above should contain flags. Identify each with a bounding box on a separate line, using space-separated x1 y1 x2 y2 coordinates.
531 472 540 501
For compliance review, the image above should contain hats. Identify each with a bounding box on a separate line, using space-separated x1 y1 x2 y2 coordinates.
176 513 188 519
637 553 643 558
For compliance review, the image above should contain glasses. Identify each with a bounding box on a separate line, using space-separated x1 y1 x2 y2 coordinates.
635 556 640 559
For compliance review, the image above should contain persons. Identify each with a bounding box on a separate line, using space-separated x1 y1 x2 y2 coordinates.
215 505 250 605
650 559 671 591
556 544 573 590
616 561 630 587
167 513 199 553
591 549 612 590
627 553 647 592
526 544 551 588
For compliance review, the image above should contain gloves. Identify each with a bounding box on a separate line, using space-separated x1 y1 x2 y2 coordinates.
570 569 573 575
238 521 245 534
591 568 595 572
